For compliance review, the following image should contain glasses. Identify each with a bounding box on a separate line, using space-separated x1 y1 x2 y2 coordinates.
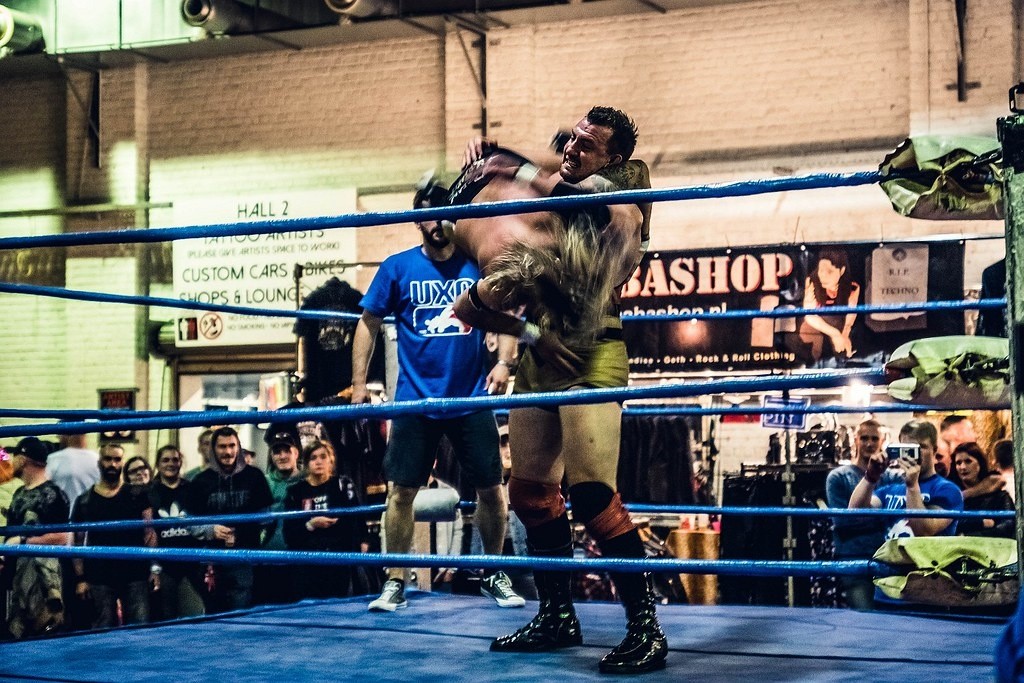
103 456 121 462
859 436 879 442
955 459 974 465
128 465 146 474
274 432 291 439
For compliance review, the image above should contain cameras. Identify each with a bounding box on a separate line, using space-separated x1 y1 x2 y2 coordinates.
886 444 921 464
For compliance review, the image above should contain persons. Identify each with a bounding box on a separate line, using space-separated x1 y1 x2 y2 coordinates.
0 410 1024 682
459 106 669 675
790 248 862 367
979 253 1009 341
351 188 525 612
444 149 617 389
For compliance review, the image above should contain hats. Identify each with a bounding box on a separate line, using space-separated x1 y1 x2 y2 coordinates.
269 432 297 449
241 449 256 458
3 437 48 464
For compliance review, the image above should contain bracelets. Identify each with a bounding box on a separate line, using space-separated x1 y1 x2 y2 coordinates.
497 360 516 376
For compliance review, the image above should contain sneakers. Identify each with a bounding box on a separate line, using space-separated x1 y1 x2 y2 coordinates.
480 571 526 608
368 580 407 612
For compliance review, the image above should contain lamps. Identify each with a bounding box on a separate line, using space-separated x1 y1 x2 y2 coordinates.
0 4 43 55
325 0 401 26
180 0 276 35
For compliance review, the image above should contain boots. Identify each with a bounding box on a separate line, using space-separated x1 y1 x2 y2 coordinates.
489 542 583 652
597 555 669 674
